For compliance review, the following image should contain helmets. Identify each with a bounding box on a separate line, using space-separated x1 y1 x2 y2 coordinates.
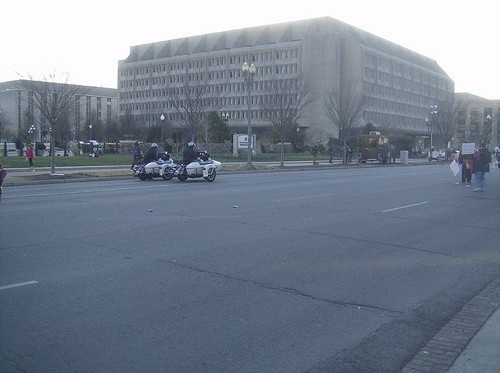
188 142 195 146
151 143 158 148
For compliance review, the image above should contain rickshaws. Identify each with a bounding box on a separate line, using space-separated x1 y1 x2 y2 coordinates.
131 151 221 182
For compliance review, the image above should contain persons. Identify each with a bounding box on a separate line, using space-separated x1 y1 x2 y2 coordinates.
329 144 334 163
458 140 490 191
26 146 34 168
142 143 159 165
184 142 197 163
131 141 142 169
347 145 353 162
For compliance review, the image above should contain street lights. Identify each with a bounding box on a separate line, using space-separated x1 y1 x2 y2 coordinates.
241 62 257 165
89 124 92 140
160 114 166 144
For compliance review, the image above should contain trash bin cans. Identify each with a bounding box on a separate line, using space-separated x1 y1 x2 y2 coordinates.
400 151 409 163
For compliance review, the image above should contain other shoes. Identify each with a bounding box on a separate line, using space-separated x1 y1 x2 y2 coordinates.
480 188 484 192
473 188 481 192
